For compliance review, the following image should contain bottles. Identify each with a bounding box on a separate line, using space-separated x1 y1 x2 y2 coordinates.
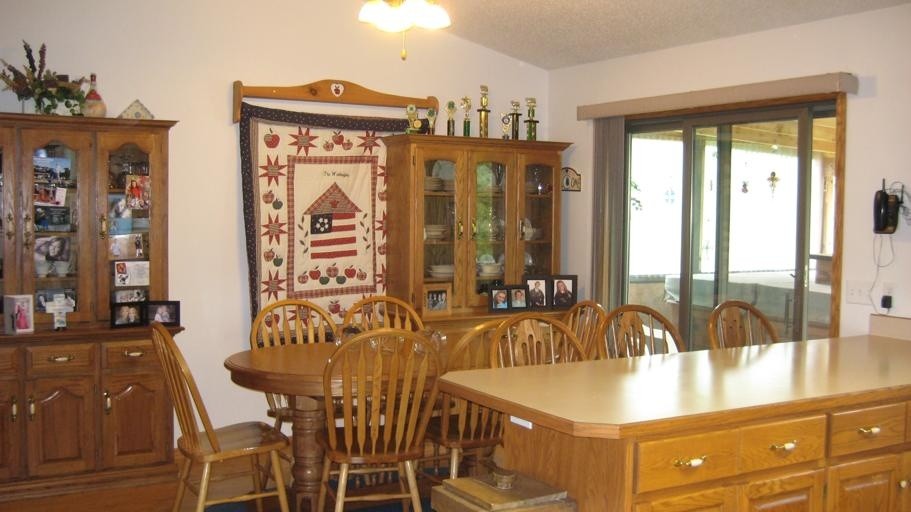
80 71 106 120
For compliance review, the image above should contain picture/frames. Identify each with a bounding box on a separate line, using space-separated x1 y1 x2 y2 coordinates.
491 275 578 311
110 301 145 329
146 301 181 326
3 294 35 336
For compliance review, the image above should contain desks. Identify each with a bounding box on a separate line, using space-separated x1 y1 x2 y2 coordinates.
430 335 911 512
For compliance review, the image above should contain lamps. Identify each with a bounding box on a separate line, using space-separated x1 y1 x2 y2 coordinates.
357 0 454 61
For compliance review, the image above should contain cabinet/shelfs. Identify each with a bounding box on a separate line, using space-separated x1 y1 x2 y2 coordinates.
0 111 185 510
380 135 575 424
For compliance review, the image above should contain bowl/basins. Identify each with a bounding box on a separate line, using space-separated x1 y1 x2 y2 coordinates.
369 335 405 353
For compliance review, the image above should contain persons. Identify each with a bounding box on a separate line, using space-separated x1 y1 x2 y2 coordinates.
46 237 69 262
128 308 139 323
511 290 525 307
34 238 50 261
161 306 170 321
128 180 139 198
15 303 29 329
492 290 506 308
139 199 149 208
554 281 571 305
110 239 121 259
529 281 544 306
109 199 127 217
127 198 136 208
133 236 142 257
154 307 162 321
36 295 45 307
115 306 128 324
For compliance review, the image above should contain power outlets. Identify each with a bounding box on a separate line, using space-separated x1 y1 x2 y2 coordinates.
882 280 896 309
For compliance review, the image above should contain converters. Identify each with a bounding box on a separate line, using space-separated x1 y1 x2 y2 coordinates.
880 295 892 308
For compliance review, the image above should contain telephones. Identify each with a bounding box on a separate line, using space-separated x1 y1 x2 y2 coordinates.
872 178 904 234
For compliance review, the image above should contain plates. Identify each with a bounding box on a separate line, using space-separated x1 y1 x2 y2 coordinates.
424 250 536 279
424 223 544 242
422 175 548 195
